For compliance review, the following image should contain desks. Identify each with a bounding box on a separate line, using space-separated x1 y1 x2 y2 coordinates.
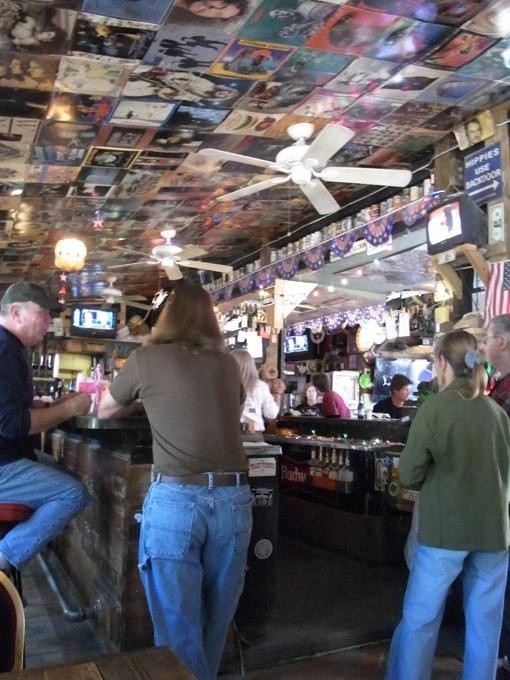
0 645 208 680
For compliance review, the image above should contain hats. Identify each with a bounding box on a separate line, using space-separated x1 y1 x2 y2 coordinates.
1 280 64 310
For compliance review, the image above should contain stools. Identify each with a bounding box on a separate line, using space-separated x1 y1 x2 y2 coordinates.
0 503 32 609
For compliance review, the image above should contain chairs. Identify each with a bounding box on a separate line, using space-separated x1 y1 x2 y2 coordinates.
1 570 26 672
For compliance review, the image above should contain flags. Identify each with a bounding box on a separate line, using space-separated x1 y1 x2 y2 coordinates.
482 259 509 322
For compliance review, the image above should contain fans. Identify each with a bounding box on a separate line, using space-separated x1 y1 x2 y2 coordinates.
197 122 413 236
68 277 153 311
107 230 233 281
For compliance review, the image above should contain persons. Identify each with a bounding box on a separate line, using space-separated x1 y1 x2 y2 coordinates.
371 372 417 421
2 1 509 273
312 373 354 418
229 346 287 441
79 309 96 330
383 328 509 680
291 382 321 416
96 277 253 680
478 313 509 673
0 278 94 607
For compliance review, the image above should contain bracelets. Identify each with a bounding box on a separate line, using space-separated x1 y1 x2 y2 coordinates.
272 391 283 396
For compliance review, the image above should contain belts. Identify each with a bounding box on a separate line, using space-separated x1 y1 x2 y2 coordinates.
154 473 249 486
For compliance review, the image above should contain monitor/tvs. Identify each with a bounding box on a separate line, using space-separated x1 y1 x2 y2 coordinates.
425 194 487 256
284 333 310 357
71 303 119 338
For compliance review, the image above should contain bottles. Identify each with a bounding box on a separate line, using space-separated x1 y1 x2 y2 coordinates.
30 341 54 378
308 446 355 494
